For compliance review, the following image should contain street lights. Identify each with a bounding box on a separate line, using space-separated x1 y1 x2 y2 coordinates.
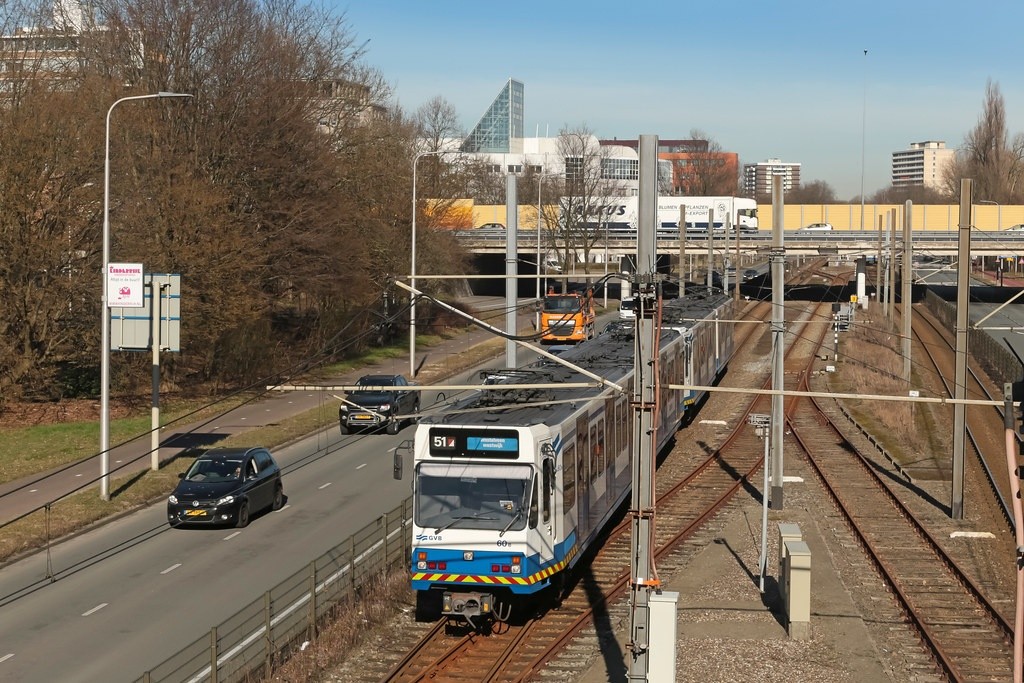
980 200 999 229
536 173 573 330
412 148 461 378
101 92 194 501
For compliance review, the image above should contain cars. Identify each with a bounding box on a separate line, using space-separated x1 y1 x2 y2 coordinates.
728 267 758 282
940 262 951 272
1004 224 1024 235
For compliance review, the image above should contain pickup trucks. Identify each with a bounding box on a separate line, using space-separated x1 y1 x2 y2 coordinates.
339 374 421 435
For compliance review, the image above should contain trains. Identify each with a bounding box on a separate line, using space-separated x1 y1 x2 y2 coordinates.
393 288 735 630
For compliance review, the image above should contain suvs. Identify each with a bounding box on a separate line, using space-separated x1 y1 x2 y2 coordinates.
167 446 283 528
799 223 832 235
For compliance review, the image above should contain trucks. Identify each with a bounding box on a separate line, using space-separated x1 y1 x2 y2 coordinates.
536 288 594 344
617 297 637 327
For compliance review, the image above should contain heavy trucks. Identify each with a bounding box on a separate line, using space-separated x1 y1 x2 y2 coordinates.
558 195 758 240
